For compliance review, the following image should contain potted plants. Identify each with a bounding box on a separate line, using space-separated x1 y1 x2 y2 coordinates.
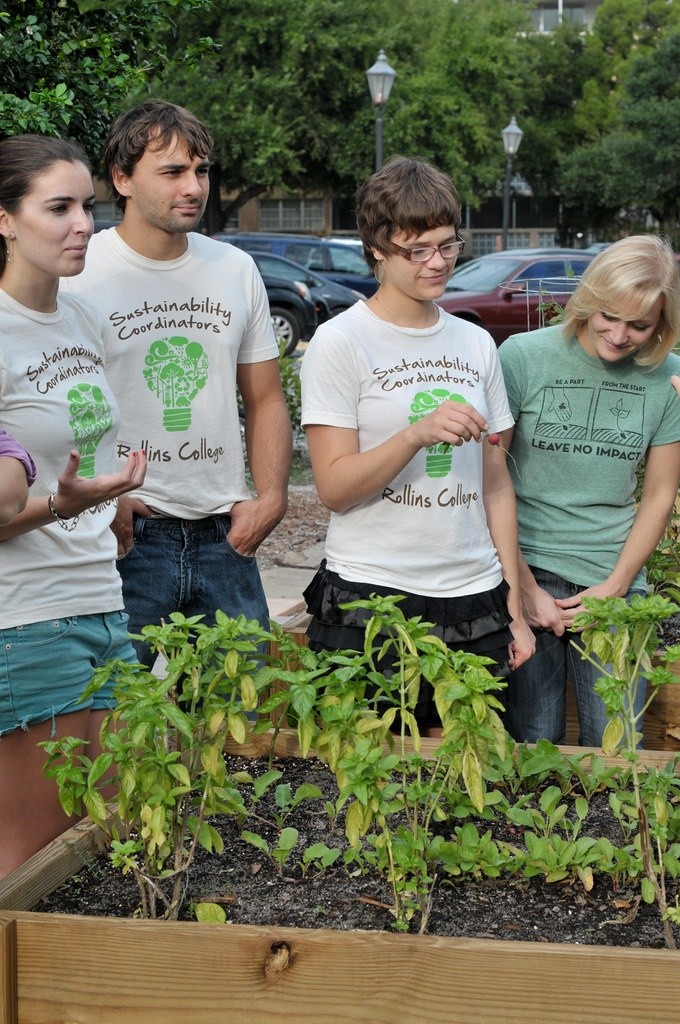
0 579 680 1024
269 458 680 751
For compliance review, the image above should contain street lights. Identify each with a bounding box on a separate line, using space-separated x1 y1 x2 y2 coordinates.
501 116 524 252
365 49 396 174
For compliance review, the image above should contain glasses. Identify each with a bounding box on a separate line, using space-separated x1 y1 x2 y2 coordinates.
382 232 466 262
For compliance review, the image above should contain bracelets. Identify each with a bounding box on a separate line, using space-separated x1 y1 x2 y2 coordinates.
50 491 79 532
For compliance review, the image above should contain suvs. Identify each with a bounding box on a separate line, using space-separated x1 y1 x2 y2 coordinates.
431 243 598 348
211 232 379 360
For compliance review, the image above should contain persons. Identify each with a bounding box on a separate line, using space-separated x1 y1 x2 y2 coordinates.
1 429 38 526
58 98 292 722
300 159 537 740
1 134 148 883
497 236 680 749
